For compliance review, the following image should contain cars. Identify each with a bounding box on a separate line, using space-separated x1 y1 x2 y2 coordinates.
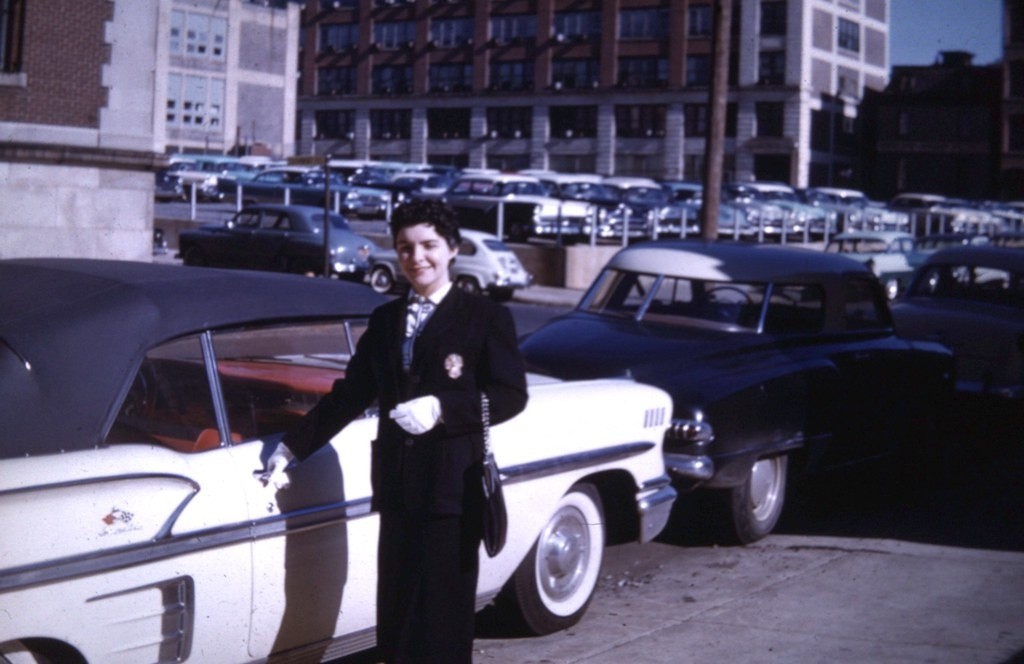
518 237 957 548
156 150 1024 303
0 258 679 664
888 244 1024 432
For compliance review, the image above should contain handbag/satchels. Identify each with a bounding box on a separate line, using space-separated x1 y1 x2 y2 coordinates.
470 457 508 558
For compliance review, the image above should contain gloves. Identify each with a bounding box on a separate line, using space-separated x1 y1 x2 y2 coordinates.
266 441 296 493
389 395 441 436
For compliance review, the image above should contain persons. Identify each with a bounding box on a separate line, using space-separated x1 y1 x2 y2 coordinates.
268 200 530 664
